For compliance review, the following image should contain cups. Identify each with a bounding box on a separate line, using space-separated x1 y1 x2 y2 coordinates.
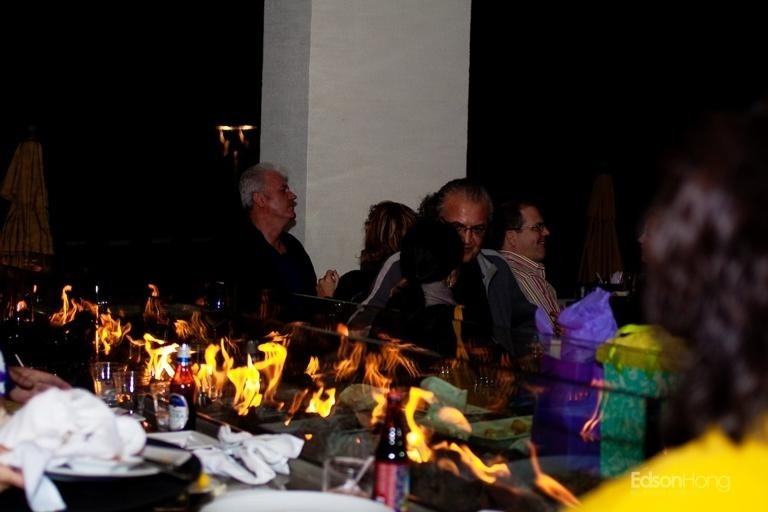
436 362 455 384
88 361 119 399
321 457 375 499
111 370 134 405
474 375 499 403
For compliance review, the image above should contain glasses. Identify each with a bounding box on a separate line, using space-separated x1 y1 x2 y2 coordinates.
516 222 543 234
444 221 486 238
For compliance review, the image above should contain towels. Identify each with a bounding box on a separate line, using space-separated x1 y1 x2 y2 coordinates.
193 426 306 486
3 388 149 509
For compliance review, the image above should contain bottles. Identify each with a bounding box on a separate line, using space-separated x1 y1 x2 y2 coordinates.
169 344 196 431
372 392 410 512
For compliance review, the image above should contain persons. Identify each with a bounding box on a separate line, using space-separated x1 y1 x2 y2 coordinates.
492 198 561 335
318 200 419 303
369 212 462 367
563 170 764 510
189 161 316 314
347 178 537 375
0 352 73 494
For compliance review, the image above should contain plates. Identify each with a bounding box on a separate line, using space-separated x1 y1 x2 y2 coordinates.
8 444 191 477
146 430 219 450
198 489 396 512
469 415 533 441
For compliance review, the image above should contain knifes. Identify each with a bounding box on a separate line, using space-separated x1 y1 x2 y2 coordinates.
133 453 196 482
229 453 257 477
